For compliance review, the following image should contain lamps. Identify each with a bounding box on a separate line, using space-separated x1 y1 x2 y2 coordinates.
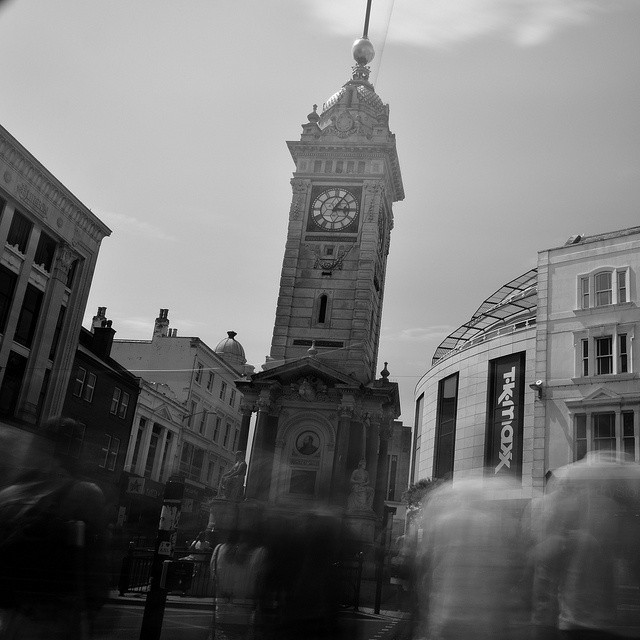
529 380 543 399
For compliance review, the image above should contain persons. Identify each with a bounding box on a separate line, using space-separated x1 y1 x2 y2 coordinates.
190 531 213 577
299 436 316 455
217 450 246 499
208 516 268 640
347 459 374 512
0 417 107 638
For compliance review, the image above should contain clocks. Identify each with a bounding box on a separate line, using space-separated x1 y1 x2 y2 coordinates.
310 187 359 232
